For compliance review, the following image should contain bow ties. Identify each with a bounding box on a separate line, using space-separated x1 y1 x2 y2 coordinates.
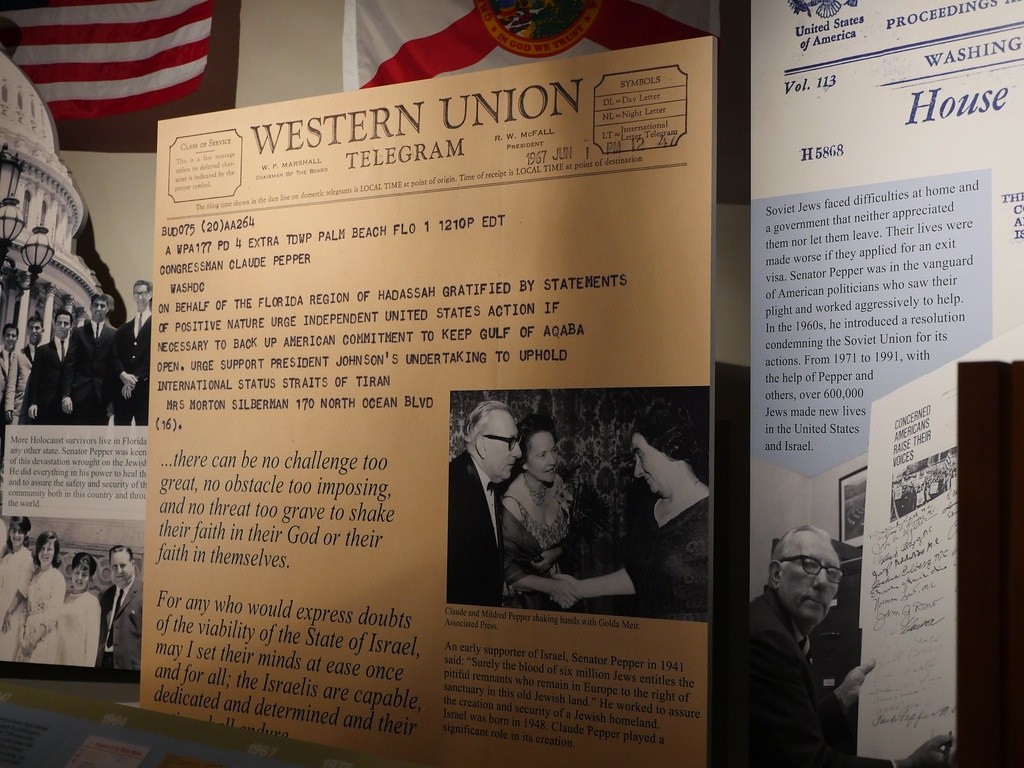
487 481 496 494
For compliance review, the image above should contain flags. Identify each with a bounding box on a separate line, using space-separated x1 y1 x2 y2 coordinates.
0 0 214 121
944 452 951 467
342 0 722 92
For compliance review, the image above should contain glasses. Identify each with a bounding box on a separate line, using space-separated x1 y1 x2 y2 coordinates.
133 291 148 298
780 555 845 583
483 433 522 451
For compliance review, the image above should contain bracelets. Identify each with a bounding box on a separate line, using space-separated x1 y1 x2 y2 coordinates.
62 397 71 403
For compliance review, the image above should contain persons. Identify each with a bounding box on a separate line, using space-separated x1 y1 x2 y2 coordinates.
0 281 152 426
447 402 583 611
750 523 953 768
0 516 147 668
551 405 709 616
892 464 956 516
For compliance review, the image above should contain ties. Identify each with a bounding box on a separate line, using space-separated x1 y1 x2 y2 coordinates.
61 340 65 362
96 323 99 340
138 314 142 333
107 589 123 648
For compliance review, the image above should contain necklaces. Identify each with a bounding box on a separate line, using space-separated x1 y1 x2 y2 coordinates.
524 472 548 506
654 479 699 523
